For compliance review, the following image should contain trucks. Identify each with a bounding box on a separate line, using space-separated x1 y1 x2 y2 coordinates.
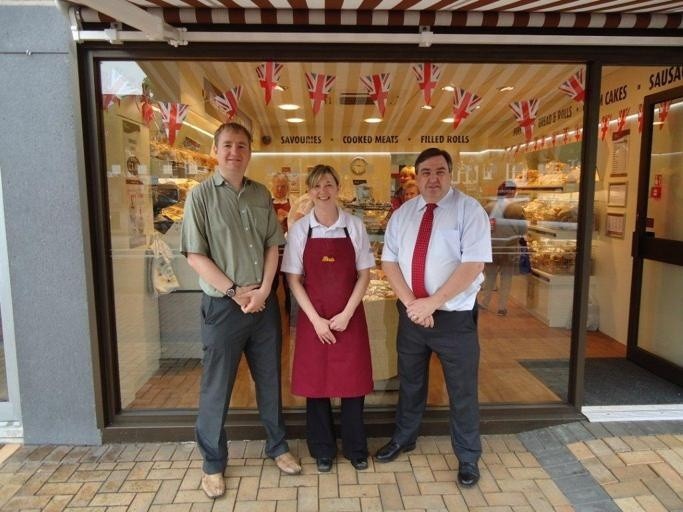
146 176 199 206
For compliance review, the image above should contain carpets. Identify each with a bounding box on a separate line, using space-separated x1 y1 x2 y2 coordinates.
512 356 681 405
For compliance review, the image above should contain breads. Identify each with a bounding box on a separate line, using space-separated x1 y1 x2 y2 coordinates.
149 138 217 224
506 161 598 276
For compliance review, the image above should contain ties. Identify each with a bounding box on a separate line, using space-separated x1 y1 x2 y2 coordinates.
411 204 438 298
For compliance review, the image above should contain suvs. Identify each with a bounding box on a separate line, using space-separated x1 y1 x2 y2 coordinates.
478 194 582 263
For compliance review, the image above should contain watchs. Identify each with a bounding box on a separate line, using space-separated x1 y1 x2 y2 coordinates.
226 282 237 298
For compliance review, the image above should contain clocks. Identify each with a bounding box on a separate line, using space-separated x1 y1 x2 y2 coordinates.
350 156 369 176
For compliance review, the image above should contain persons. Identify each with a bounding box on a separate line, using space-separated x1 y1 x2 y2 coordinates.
279 163 378 472
180 122 303 499
478 179 528 317
374 148 492 488
270 167 420 326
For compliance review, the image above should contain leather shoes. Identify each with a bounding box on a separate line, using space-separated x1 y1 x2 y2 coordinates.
316 456 333 471
372 438 416 462
350 455 368 470
273 450 303 475
458 460 479 487
200 470 226 498
